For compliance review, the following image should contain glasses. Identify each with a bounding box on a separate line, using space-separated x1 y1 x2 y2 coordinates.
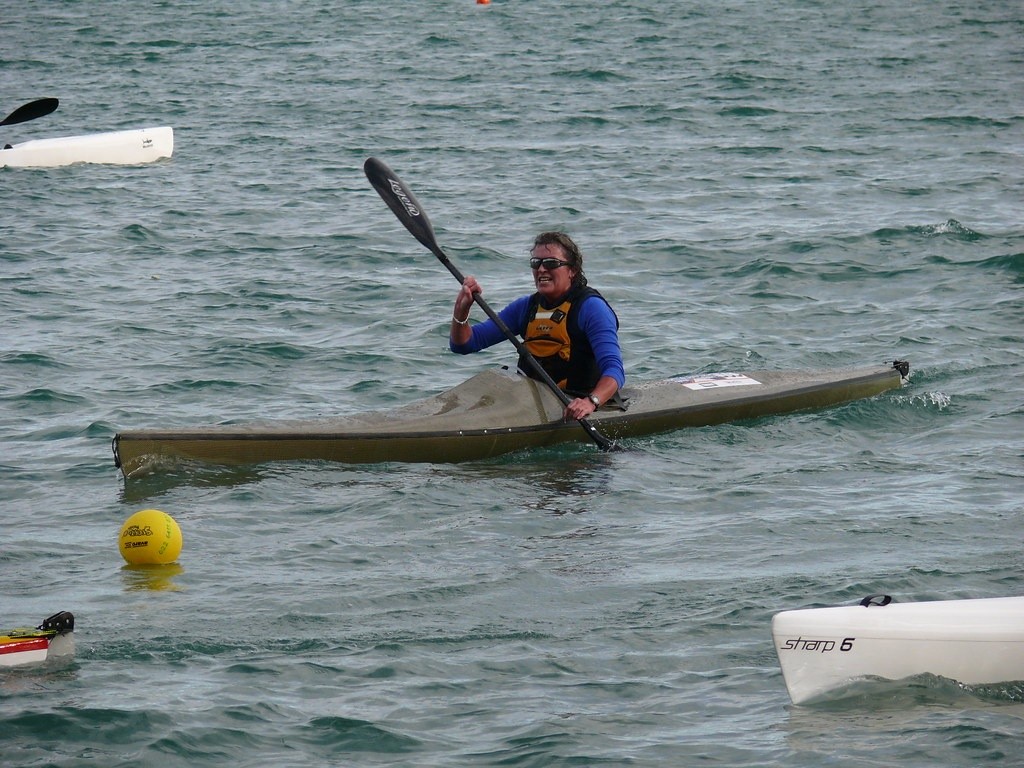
530 257 573 269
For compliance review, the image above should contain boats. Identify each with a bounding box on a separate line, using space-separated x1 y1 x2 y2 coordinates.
0 611 76 667
114 358 911 477
771 593 1024 708
0 125 174 169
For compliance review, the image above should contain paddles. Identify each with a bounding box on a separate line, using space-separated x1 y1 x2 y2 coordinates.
362 156 624 448
0 97 60 127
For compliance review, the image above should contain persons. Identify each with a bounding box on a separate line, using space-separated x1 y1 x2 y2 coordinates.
450 233 625 419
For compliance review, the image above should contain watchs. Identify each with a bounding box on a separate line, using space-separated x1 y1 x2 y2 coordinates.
584 394 600 412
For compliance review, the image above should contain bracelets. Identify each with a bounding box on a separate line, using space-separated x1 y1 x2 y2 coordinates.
453 313 470 324
